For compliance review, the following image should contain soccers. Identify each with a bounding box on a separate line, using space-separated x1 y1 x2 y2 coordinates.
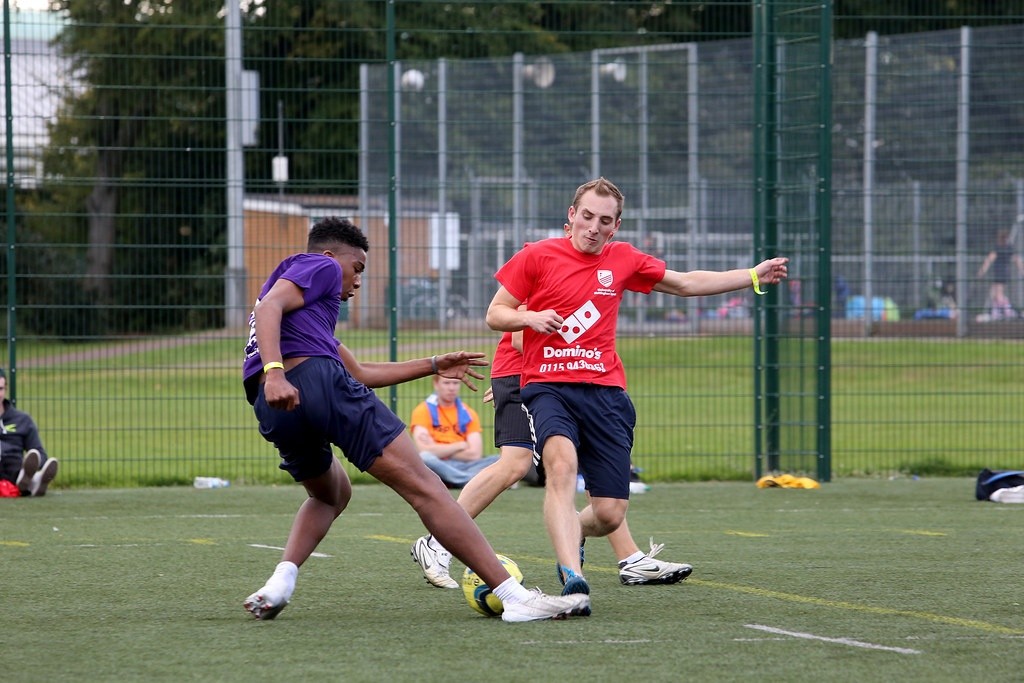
461 554 523 617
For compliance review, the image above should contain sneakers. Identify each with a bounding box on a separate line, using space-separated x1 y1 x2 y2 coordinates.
501 586 582 621
243 580 293 620
619 536 693 584
409 533 460 589
561 567 590 615
557 510 585 586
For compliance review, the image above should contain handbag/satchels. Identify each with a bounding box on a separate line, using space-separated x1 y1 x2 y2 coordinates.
975 469 1024 500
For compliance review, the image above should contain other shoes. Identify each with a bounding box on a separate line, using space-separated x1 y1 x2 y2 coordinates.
16 449 41 489
31 457 58 497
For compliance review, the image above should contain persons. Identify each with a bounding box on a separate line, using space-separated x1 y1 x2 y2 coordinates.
486 179 789 597
411 296 693 589
928 276 957 318
0 368 58 497
979 230 1024 319
412 375 501 484
243 216 582 622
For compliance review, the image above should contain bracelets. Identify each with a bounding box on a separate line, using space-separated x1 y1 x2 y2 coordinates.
749 268 768 295
264 362 284 374
